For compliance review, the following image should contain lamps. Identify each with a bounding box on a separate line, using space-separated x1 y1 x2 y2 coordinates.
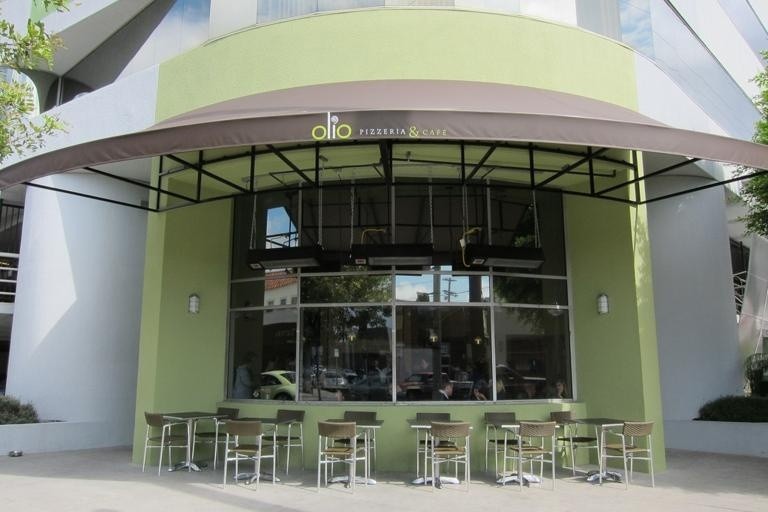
347 168 434 267
475 336 482 344
430 334 438 342
246 172 323 270
596 293 609 313
347 333 356 342
461 164 545 269
189 293 200 313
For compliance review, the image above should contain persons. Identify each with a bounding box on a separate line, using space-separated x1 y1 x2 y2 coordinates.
473 378 507 400
437 379 455 401
552 378 568 399
232 350 263 399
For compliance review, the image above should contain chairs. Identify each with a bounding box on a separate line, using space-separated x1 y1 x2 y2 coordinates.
484 412 525 474
431 422 474 488
142 411 192 476
508 421 556 490
193 407 239 470
262 408 305 474
317 421 368 489
416 413 455 479
602 421 656 490
548 411 600 476
335 409 376 479
223 419 273 484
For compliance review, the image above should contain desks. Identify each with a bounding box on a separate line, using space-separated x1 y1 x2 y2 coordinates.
495 423 560 482
409 422 472 485
327 419 384 484
569 418 625 477
162 412 228 472
232 417 296 482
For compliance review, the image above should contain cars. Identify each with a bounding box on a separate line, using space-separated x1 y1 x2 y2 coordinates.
254 360 551 401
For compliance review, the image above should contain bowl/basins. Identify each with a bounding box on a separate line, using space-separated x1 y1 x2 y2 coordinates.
9 451 23 457
